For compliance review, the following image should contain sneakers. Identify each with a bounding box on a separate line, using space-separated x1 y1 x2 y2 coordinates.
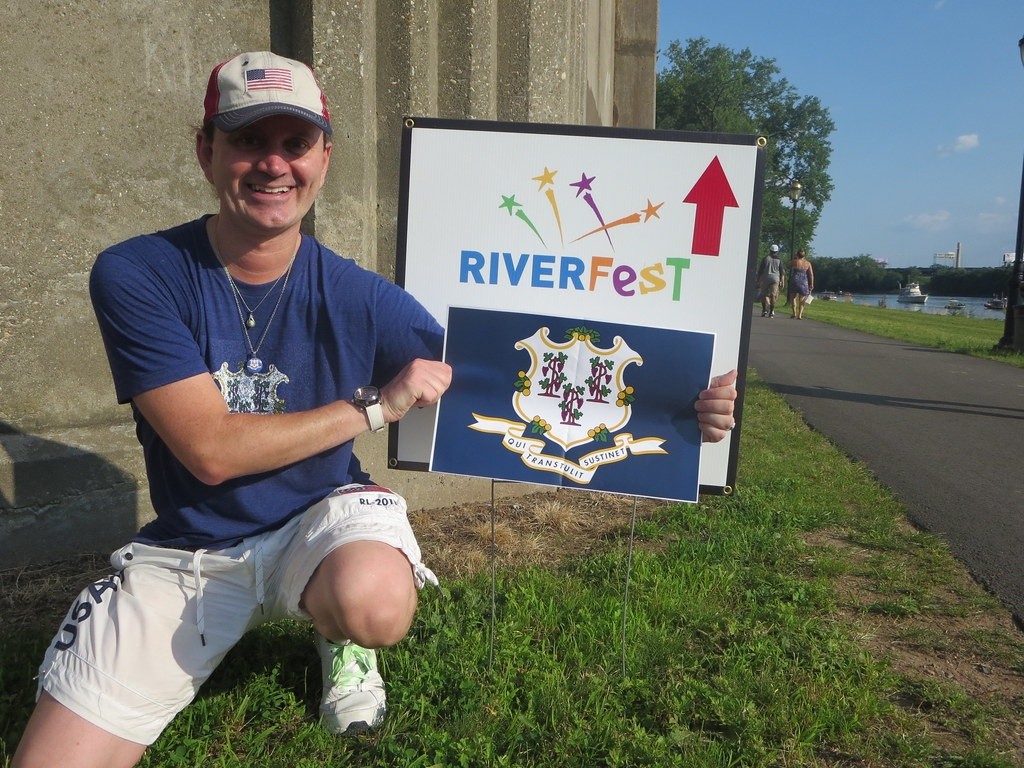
316 629 387 733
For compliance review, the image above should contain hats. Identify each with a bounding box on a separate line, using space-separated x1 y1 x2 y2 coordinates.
204 51 333 135
770 244 779 252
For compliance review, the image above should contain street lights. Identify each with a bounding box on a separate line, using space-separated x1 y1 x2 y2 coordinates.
785 179 805 307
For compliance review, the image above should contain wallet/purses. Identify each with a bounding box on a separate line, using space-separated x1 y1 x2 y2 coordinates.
805 295 814 305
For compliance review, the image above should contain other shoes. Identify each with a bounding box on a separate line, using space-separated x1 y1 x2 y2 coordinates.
798 315 802 319
791 314 796 318
761 311 767 317
769 313 774 317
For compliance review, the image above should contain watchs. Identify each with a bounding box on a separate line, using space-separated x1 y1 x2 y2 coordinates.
351 384 385 434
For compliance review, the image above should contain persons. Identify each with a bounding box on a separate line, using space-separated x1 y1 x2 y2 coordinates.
786 248 814 319
9 50 738 768
756 244 785 318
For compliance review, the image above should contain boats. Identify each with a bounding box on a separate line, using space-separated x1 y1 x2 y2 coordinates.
985 287 1009 311
838 291 843 295
844 292 851 296
944 299 966 309
817 289 837 303
896 280 928 305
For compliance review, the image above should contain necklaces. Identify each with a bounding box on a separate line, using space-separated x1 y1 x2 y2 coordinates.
214 229 296 374
